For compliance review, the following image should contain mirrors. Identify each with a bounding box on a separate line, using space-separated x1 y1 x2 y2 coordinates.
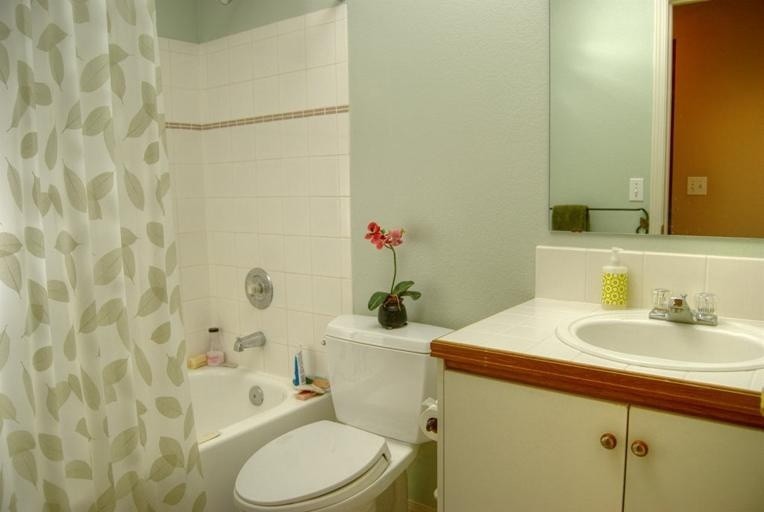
547 0 764 237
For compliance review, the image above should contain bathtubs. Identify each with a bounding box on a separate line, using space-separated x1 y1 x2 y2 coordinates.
185 361 337 512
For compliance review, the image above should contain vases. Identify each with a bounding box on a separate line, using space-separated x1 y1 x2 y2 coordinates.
378 303 409 327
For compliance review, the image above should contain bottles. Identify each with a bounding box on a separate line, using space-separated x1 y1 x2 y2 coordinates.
206 327 224 366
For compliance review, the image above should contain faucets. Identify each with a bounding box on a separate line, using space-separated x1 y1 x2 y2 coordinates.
666 296 693 324
233 331 266 353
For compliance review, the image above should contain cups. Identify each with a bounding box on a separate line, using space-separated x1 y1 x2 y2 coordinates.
696 292 718 322
652 288 670 314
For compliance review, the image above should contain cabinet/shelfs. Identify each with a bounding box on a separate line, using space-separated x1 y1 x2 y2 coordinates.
438 360 764 512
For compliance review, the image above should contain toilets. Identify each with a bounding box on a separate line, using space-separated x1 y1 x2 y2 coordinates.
232 314 456 512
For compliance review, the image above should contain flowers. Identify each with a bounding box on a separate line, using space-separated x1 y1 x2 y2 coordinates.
364 222 420 311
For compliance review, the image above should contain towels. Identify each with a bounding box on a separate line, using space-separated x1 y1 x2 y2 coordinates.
551 204 590 232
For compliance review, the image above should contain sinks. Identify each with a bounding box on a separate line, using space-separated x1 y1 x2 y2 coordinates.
556 308 764 372
